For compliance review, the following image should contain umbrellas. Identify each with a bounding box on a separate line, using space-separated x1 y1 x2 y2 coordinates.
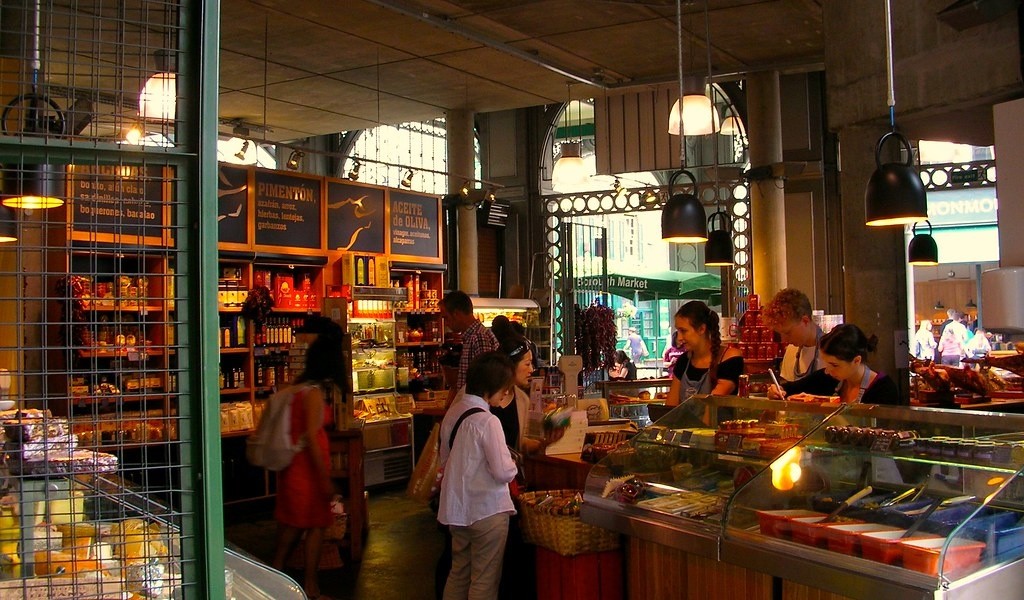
553 266 722 394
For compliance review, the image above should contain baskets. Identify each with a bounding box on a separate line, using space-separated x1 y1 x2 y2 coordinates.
518 489 622 556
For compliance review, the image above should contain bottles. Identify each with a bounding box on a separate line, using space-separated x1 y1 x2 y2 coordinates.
350 276 443 390
357 258 363 284
368 259 374 286
73 270 319 396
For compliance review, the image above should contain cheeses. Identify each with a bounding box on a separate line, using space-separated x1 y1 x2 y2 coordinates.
0 492 182 600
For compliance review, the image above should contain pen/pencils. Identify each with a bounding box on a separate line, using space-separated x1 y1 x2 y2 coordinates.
768 369 785 401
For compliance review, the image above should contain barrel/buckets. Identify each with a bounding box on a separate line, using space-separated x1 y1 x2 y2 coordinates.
33 520 164 597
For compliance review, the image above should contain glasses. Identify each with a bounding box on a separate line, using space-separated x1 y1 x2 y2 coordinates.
509 336 532 358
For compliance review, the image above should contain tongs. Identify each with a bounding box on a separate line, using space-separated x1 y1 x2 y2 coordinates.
878 483 927 508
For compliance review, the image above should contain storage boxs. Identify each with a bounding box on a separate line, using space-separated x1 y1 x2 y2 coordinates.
758 491 1024 581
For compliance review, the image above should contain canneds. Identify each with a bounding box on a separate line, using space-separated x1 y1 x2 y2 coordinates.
913 436 996 465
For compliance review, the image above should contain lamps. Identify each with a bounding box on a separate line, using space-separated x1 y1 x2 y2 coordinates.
349 160 361 179
863 0 929 227
614 178 658 203
137 1 177 120
720 109 745 136
909 138 940 265
401 169 414 187
661 0 707 243
668 2 720 136
458 180 471 197
552 82 589 187
286 149 302 170
234 141 248 159
965 264 977 308
485 187 497 201
705 0 736 266
934 266 944 309
2 0 64 210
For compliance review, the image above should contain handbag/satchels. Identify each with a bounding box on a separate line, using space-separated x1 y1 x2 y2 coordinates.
405 423 445 502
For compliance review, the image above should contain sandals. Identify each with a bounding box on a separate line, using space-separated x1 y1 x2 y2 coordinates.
306 591 337 600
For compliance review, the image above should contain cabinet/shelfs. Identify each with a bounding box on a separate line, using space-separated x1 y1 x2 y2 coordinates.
583 394 1023 587
66 252 443 453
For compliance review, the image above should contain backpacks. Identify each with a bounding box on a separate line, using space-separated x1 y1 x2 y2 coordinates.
246 381 325 471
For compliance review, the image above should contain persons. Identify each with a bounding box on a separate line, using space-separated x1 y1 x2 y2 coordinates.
274 328 351 600
491 315 540 377
916 320 938 362
663 301 744 478
663 330 687 377
429 334 564 600
623 326 649 366
438 291 499 395
762 286 828 422
435 350 520 600
936 308 992 369
607 348 639 415
817 321 933 487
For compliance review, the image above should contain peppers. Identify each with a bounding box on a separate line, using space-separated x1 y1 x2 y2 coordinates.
573 303 619 376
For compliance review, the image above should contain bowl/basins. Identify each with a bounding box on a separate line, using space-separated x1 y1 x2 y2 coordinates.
0 401 15 411
